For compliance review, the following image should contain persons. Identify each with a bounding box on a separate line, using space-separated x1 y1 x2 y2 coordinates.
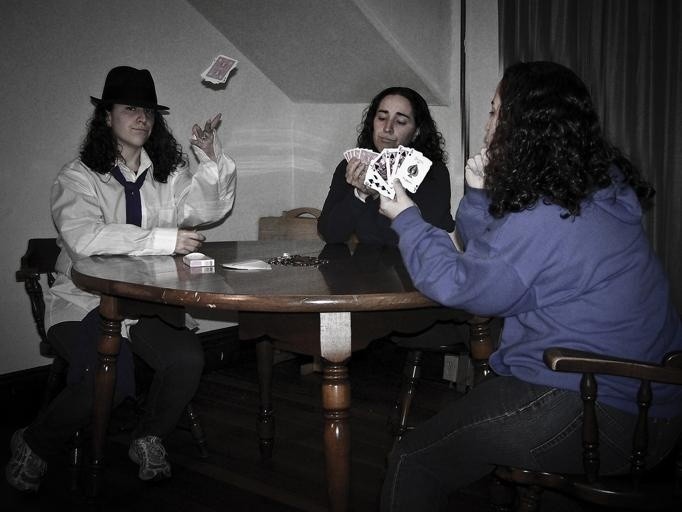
3 64 239 496
313 83 456 252
360 60 680 506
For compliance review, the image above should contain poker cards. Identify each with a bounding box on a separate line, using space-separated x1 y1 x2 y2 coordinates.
201 55 238 84
343 145 433 200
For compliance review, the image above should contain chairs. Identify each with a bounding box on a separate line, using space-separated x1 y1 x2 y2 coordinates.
14 237 211 491
388 322 470 451
493 348 682 512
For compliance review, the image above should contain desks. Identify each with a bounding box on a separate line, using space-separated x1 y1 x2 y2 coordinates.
70 241 495 512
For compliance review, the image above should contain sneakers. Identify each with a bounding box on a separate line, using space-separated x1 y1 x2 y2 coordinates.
10 429 44 493
127 437 170 480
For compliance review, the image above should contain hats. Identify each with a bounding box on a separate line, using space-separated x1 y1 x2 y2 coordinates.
91 65 170 111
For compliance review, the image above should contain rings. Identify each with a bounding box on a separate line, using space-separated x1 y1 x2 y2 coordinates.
199 133 207 143
